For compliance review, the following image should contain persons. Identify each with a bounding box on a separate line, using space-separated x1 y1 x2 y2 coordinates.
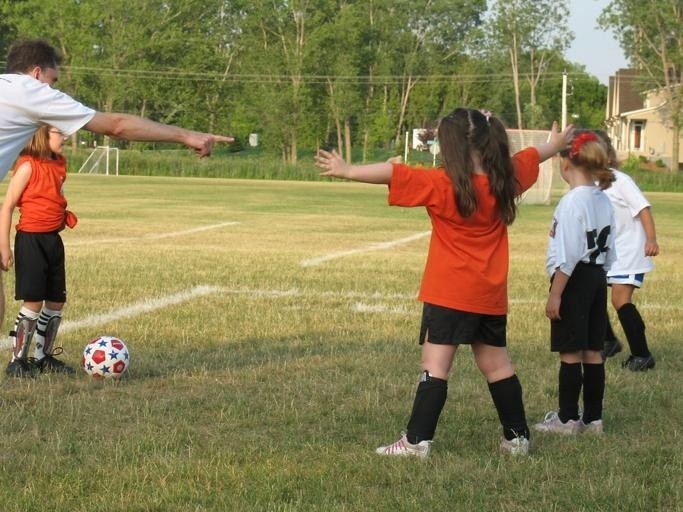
0 36 235 331
0 123 75 378
531 127 618 436
593 129 660 373
313 106 575 462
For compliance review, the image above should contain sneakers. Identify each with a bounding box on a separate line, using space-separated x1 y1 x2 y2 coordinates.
497 436 531 460
33 357 76 375
621 353 655 375
531 414 580 435
5 359 43 378
574 415 605 438
376 434 432 461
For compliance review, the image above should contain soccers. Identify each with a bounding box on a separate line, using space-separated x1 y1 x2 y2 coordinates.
83 336 129 380
